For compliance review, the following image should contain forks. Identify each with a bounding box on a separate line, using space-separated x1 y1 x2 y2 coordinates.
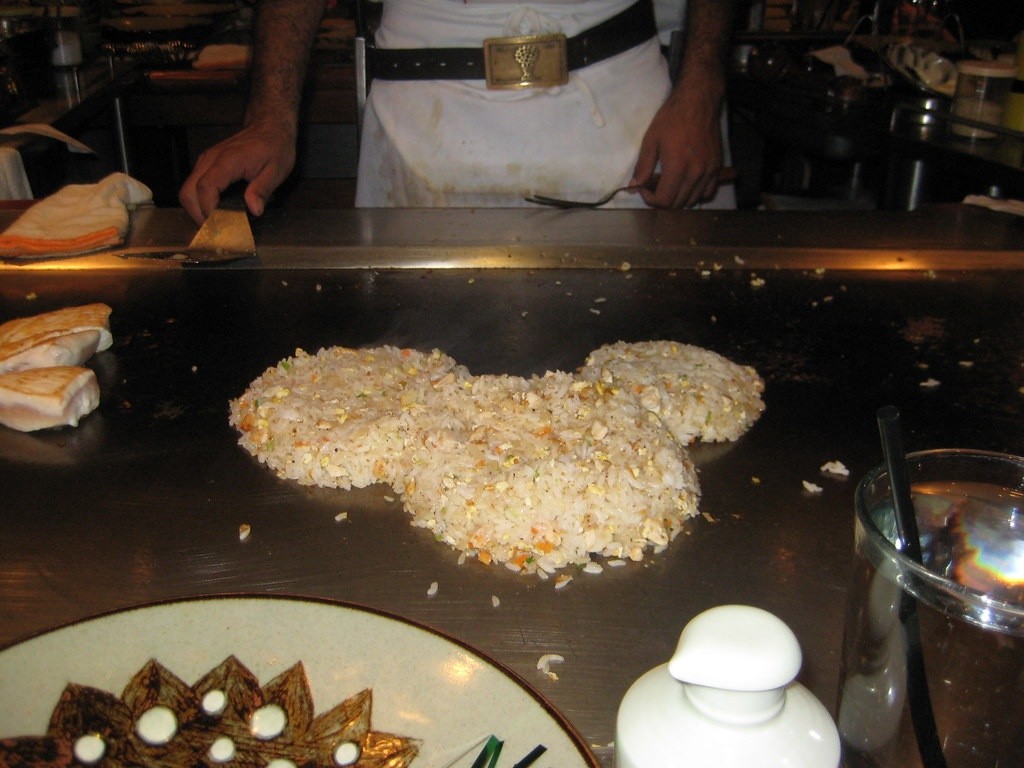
525 167 737 209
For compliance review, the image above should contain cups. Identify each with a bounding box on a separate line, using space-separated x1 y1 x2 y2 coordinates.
835 448 1024 768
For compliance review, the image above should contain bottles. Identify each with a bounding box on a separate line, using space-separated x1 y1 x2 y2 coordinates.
614 604 840 768
49 18 83 66
948 60 1017 138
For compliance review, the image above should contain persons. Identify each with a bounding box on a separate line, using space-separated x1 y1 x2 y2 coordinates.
178 0 739 226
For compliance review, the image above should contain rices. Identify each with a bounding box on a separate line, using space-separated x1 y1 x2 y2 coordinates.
228 338 767 605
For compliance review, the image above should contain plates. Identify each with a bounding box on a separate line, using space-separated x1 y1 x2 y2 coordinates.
0 596 600 768
